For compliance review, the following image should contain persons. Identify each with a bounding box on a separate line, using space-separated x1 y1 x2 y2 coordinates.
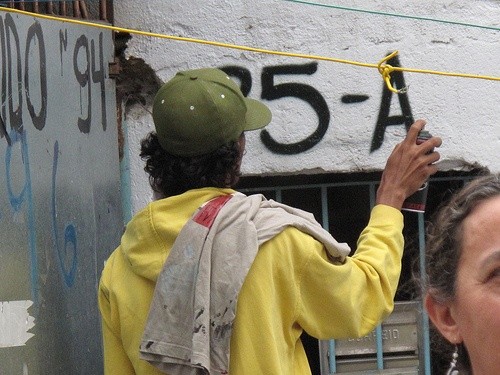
97 67 442 375
409 172 500 375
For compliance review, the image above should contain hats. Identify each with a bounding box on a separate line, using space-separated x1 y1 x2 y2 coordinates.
149 65 273 150
